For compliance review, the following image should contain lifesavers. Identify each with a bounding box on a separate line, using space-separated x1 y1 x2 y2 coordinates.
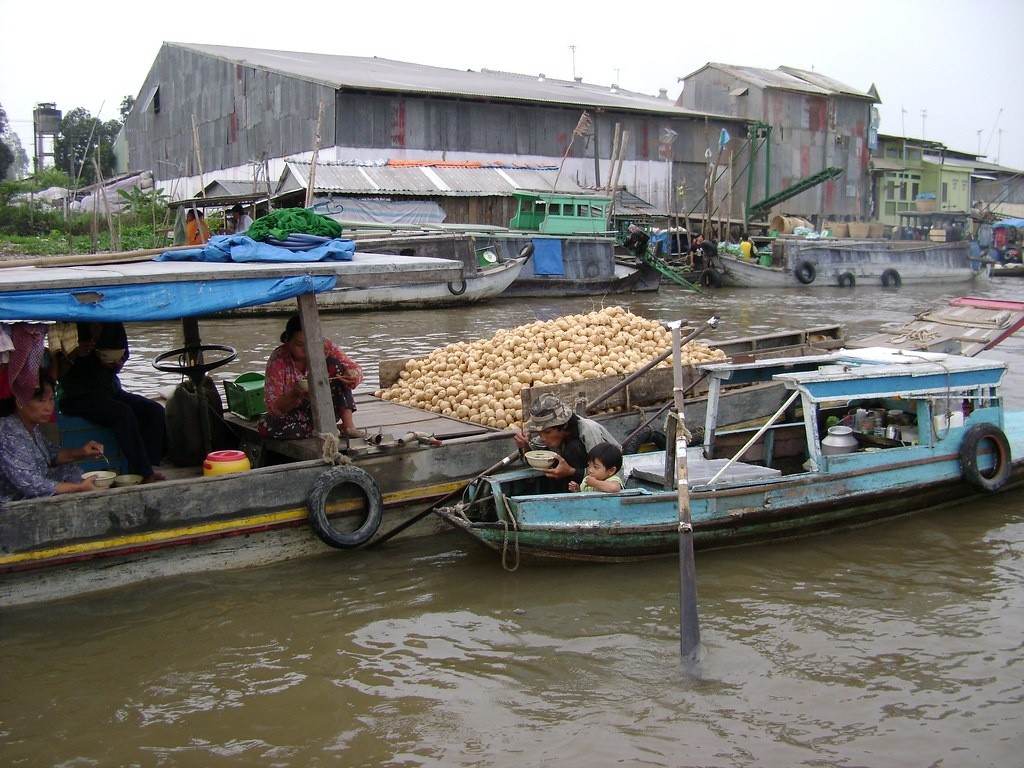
623 427 667 455
840 272 855 287
701 240 717 257
882 268 901 287
514 242 535 266
448 278 467 296
959 421 1012 492
308 465 384 549
700 268 721 289
795 260 816 284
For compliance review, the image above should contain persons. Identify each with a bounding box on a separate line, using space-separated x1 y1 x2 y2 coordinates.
48 322 166 484
689 234 704 270
755 228 771 251
185 209 208 245
739 233 760 264
257 315 365 441
513 393 622 493
568 442 624 493
0 368 110 503
219 204 254 235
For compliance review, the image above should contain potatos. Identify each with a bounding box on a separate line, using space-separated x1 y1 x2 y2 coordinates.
373 306 730 430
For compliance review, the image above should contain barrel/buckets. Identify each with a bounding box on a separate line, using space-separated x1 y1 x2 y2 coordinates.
827 222 883 238
822 425 858 456
203 450 250 476
770 214 814 234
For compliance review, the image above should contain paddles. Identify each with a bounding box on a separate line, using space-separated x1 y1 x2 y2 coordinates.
358 313 722 551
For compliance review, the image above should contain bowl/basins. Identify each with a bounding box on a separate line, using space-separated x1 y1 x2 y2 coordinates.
524 450 557 471
114 474 143 487
297 380 308 390
81 470 117 487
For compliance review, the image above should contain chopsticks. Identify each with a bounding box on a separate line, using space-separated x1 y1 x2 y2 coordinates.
517 433 534 451
329 376 342 380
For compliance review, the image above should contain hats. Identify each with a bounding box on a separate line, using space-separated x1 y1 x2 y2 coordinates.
524 393 573 431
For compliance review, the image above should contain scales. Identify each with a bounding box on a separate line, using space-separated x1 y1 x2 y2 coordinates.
476 245 500 268
222 370 267 420
757 250 775 267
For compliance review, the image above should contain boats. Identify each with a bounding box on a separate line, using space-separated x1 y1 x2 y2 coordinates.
340 189 663 299
986 218 1024 276
0 241 1024 606
0 193 536 318
700 210 984 287
433 346 1024 565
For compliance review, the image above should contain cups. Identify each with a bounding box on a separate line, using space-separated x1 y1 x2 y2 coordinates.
856 409 866 431
